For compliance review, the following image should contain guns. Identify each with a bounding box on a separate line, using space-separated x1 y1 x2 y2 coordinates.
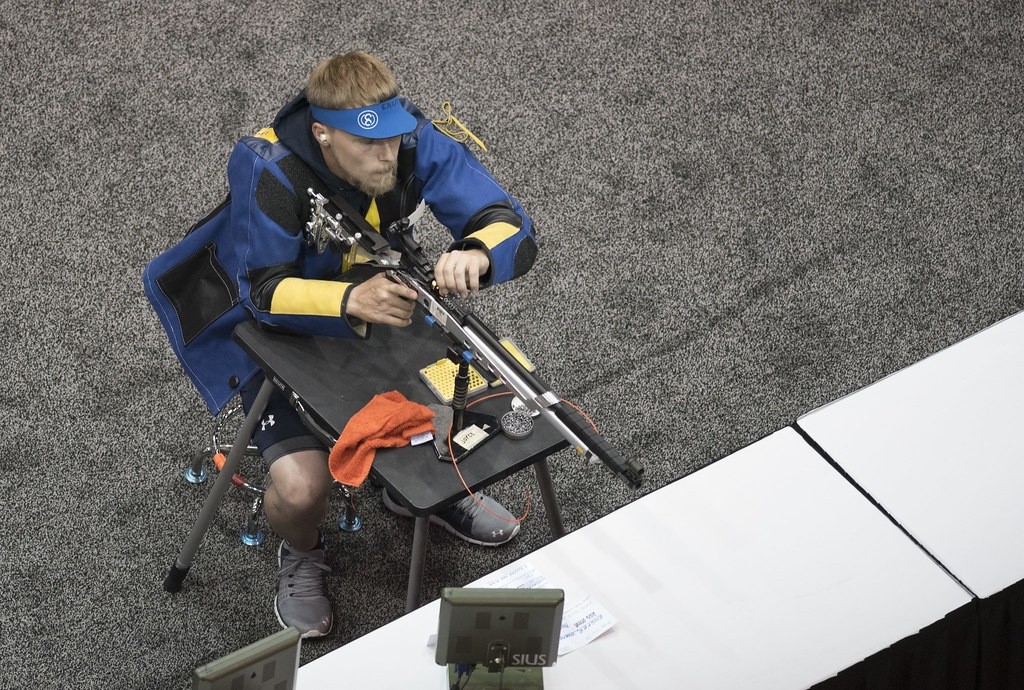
305 187 646 493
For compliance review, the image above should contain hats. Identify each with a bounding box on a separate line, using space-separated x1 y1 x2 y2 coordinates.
310 92 418 139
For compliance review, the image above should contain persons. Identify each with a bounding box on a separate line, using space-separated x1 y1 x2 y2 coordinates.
143 51 538 639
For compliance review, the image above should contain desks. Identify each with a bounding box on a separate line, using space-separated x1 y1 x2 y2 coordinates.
794 311 1024 690
293 424 979 690
164 260 571 613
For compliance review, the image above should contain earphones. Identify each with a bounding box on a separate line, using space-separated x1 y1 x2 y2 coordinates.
319 134 327 142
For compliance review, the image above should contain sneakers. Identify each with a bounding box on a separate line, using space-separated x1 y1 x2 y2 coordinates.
382 485 520 546
275 531 334 637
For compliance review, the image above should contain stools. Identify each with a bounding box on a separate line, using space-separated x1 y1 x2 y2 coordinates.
187 405 360 546
